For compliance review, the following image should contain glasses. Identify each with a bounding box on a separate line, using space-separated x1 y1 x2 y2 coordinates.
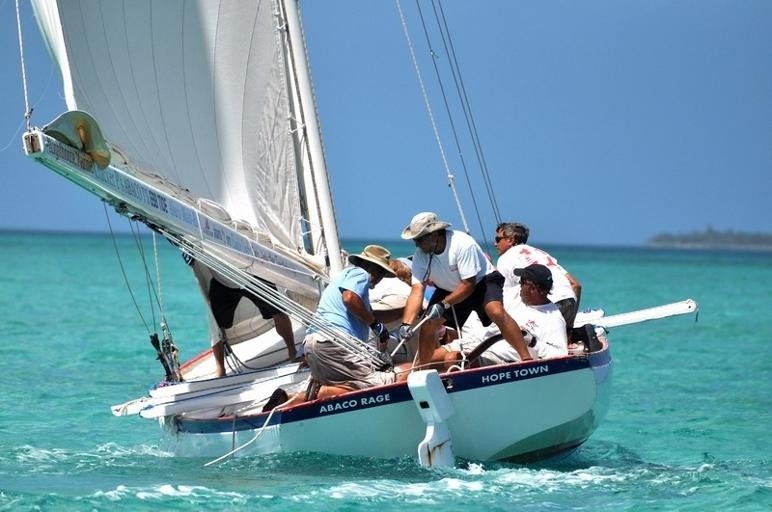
493 234 510 243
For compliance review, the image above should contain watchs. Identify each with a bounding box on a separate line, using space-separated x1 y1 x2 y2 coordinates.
441 298 451 309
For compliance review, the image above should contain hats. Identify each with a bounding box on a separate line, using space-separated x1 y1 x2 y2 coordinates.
401 211 452 240
348 243 398 280
514 263 554 289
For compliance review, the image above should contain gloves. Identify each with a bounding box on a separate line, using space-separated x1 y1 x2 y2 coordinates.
425 302 446 319
398 322 413 343
369 320 390 343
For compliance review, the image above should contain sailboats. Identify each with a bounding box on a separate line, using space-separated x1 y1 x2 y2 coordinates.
16 0 697 471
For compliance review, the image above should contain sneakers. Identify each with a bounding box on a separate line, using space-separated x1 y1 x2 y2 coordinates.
582 324 601 354
303 379 322 403
262 388 287 415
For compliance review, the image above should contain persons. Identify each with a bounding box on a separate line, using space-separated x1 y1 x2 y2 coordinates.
207 266 306 376
427 263 568 374
261 244 398 414
388 254 414 287
399 210 532 370
493 222 602 353
